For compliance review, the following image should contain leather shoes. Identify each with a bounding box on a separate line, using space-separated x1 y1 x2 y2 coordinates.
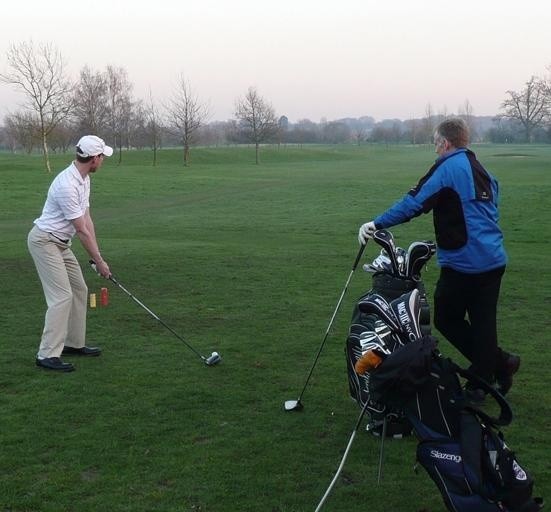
35 355 75 372
62 345 100 357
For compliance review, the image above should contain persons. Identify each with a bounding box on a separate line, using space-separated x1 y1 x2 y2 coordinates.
26 135 115 372
357 117 521 402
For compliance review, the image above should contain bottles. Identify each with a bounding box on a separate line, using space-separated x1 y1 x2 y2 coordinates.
102 288 108 305
90 293 97 308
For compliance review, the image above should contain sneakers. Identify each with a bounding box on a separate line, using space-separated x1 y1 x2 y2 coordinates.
462 382 485 403
499 353 521 396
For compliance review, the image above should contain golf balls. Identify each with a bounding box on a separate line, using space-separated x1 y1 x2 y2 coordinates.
212 352 217 357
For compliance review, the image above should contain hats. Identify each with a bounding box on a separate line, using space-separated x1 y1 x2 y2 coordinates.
75 134 113 158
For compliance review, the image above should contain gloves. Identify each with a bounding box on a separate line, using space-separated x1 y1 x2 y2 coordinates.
358 221 376 245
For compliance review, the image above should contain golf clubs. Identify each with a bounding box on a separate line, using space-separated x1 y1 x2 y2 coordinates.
362 229 438 278
89 259 221 366
359 289 422 356
285 229 374 412
